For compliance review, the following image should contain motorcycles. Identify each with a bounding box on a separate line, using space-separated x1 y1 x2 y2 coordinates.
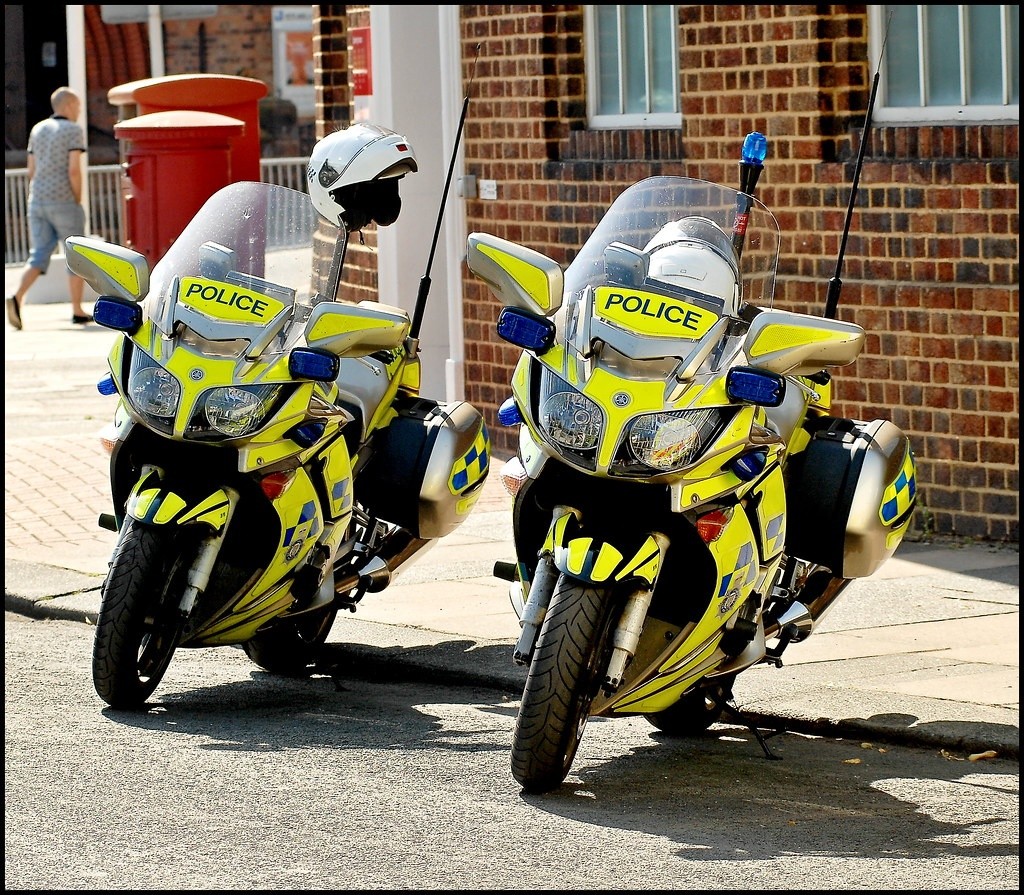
60 120 489 707
466 59 920 794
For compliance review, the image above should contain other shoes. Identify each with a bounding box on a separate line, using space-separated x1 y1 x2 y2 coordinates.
6 296 22 330
73 314 94 323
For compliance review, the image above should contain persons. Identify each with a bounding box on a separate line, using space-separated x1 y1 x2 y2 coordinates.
6 85 98 329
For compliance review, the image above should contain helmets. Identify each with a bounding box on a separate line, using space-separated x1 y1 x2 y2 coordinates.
306 121 419 234
